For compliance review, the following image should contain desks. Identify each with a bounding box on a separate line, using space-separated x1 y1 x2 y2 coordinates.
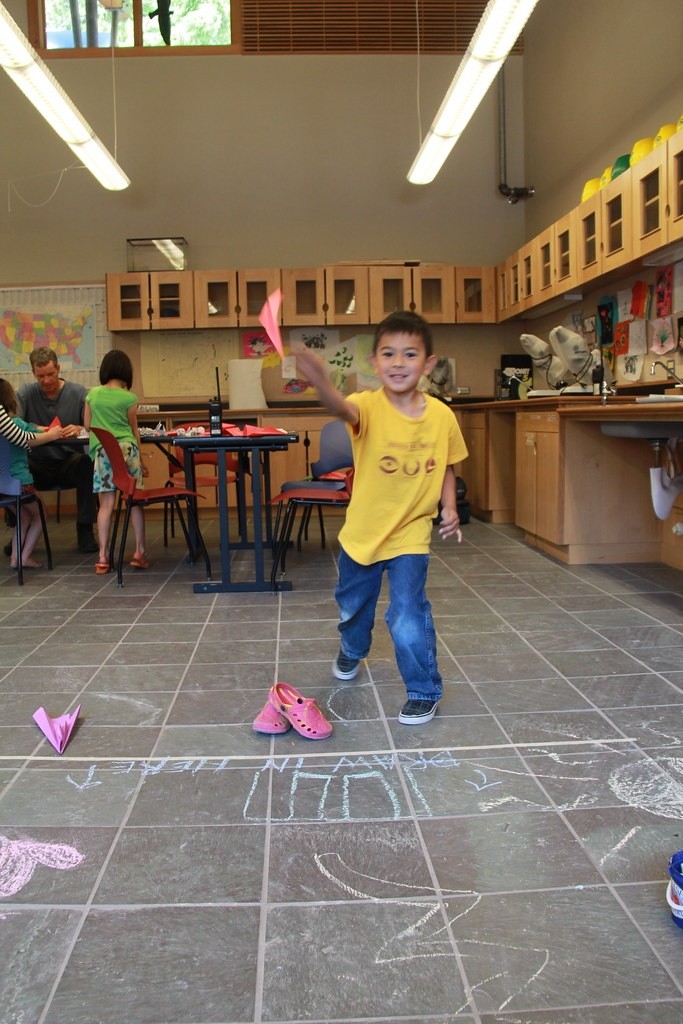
175 432 296 595
28 433 173 447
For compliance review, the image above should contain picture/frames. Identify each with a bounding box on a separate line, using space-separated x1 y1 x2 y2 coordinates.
665 359 675 379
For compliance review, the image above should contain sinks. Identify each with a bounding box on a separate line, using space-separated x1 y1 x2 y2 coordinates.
601 392 683 443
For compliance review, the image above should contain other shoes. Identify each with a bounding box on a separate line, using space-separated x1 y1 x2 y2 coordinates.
331 647 362 682
78 539 101 555
396 695 440 726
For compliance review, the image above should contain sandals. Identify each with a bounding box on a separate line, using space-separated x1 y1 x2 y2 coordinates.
94 562 110 574
130 553 151 568
252 698 292 735
267 682 334 739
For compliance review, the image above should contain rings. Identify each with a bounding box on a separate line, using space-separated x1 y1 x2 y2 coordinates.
70 432 75 437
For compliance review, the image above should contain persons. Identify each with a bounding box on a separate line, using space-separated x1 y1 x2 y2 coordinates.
289 310 469 726
0 378 64 569
10 347 100 552
84 350 149 574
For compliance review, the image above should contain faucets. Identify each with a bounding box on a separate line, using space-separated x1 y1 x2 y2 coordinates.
651 360 683 389
507 376 536 394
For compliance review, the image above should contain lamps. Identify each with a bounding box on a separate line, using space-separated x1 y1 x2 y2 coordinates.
0 0 131 192
405 0 539 187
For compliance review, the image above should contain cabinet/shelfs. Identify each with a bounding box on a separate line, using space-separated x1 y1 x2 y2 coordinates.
283 265 369 329
630 132 683 258
578 172 629 283
454 266 497 323
536 213 577 302
266 415 357 506
508 243 537 308
107 269 195 331
456 412 490 509
192 267 282 328
369 263 455 325
169 417 261 508
497 272 507 310
514 411 557 543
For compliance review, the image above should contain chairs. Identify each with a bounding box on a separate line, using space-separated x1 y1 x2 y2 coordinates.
0 418 356 589
